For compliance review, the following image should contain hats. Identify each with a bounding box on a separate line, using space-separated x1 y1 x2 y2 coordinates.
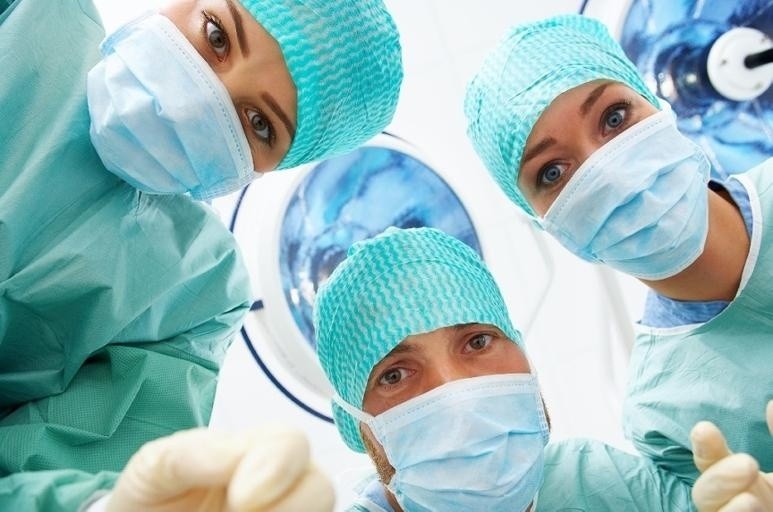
239 0 404 172
313 226 524 454
463 15 663 232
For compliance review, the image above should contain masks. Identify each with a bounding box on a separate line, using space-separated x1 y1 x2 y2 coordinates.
86 7 259 203
520 94 713 283
330 328 549 511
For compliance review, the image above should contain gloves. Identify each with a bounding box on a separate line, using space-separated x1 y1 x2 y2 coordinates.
84 426 337 511
690 398 773 512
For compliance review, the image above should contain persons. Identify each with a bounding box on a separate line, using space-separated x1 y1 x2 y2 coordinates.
462 16 773 512
0 0 403 511
312 228 696 511
